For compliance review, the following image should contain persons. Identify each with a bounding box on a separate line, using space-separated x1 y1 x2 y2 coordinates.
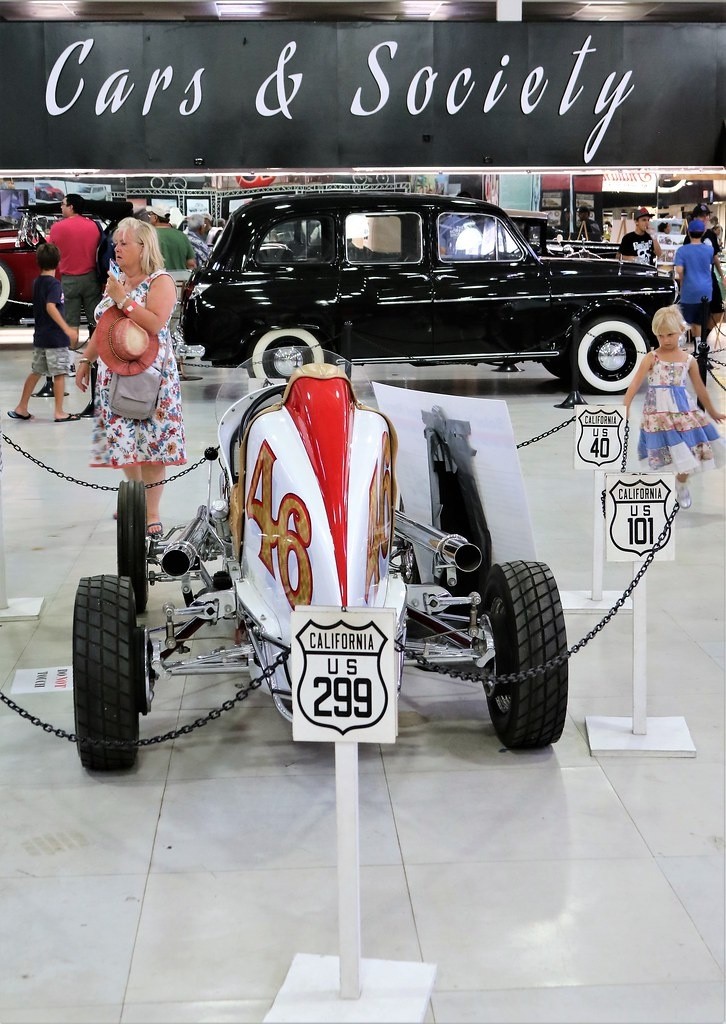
622 304 726 509
709 216 723 247
146 204 197 271
6 242 81 422
616 207 662 277
74 216 188 536
672 219 714 359
657 222 671 234
48 193 225 378
560 205 612 242
683 204 725 343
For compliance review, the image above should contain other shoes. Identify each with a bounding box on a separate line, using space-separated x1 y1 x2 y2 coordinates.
675 487 692 509
690 351 700 360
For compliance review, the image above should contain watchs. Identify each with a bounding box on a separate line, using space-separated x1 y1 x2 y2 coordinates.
48 193 101 378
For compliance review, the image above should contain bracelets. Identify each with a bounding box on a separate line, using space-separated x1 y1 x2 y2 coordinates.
117 296 129 310
124 301 137 316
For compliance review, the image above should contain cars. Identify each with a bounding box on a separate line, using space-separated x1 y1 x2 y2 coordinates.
0 200 138 323
364 205 622 260
72 347 569 771
178 191 678 395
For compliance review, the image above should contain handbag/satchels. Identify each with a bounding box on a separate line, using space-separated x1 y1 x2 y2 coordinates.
108 369 154 420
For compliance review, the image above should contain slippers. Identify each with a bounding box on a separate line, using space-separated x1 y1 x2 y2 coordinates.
54 413 80 422
8 411 35 420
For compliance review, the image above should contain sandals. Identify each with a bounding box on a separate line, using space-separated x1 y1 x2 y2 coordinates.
147 521 164 537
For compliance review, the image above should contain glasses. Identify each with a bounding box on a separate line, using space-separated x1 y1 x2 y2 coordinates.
147 212 154 218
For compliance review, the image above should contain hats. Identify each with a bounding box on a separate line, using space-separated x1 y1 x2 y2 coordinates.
687 220 705 232
146 203 171 220
692 203 712 216
633 208 655 220
577 207 590 214
95 305 159 375
